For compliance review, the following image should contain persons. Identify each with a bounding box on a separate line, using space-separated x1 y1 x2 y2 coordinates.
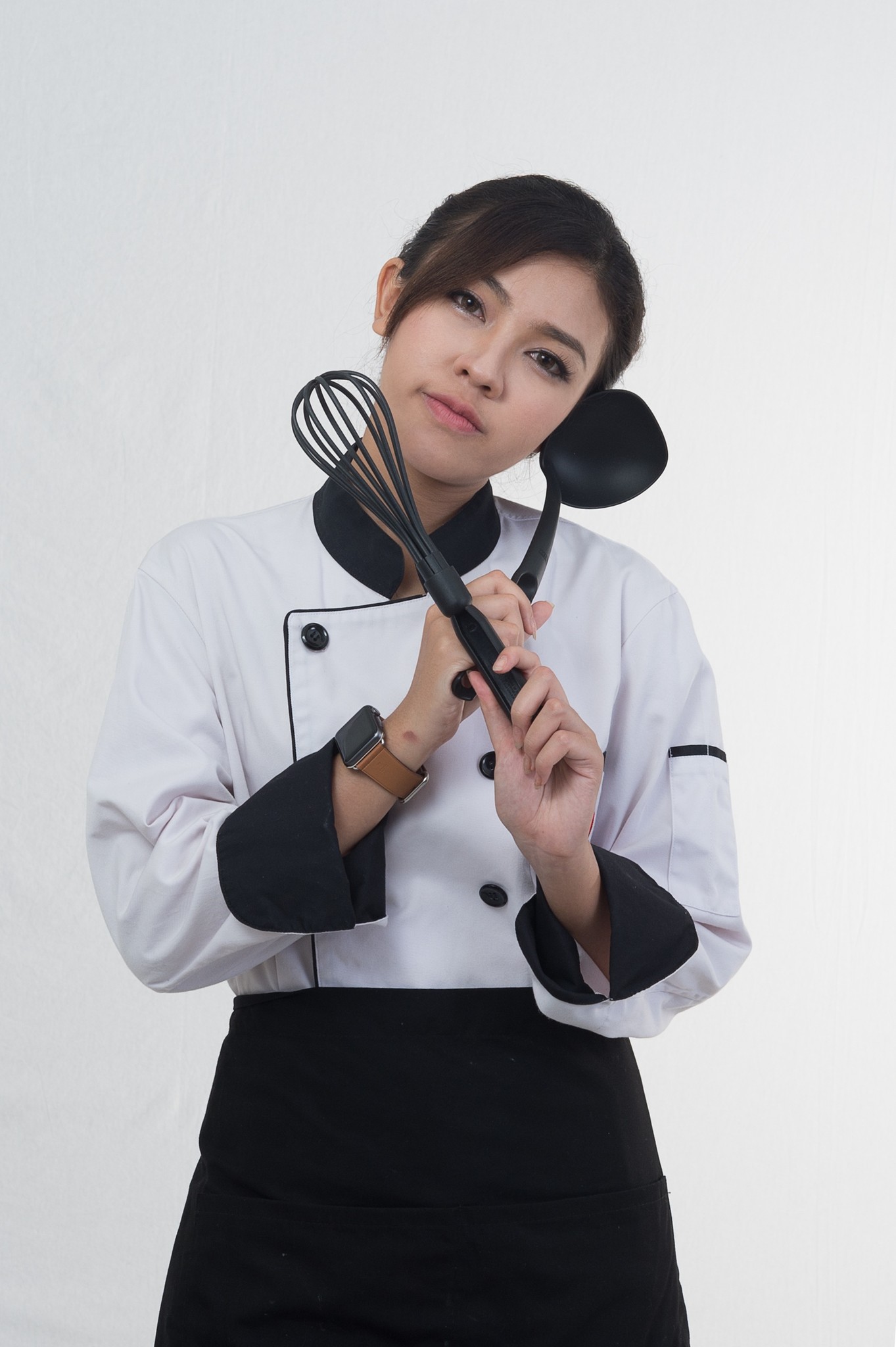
83 177 748 1347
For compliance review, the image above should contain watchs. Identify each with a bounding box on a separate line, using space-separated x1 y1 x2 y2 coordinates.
335 706 427 807
